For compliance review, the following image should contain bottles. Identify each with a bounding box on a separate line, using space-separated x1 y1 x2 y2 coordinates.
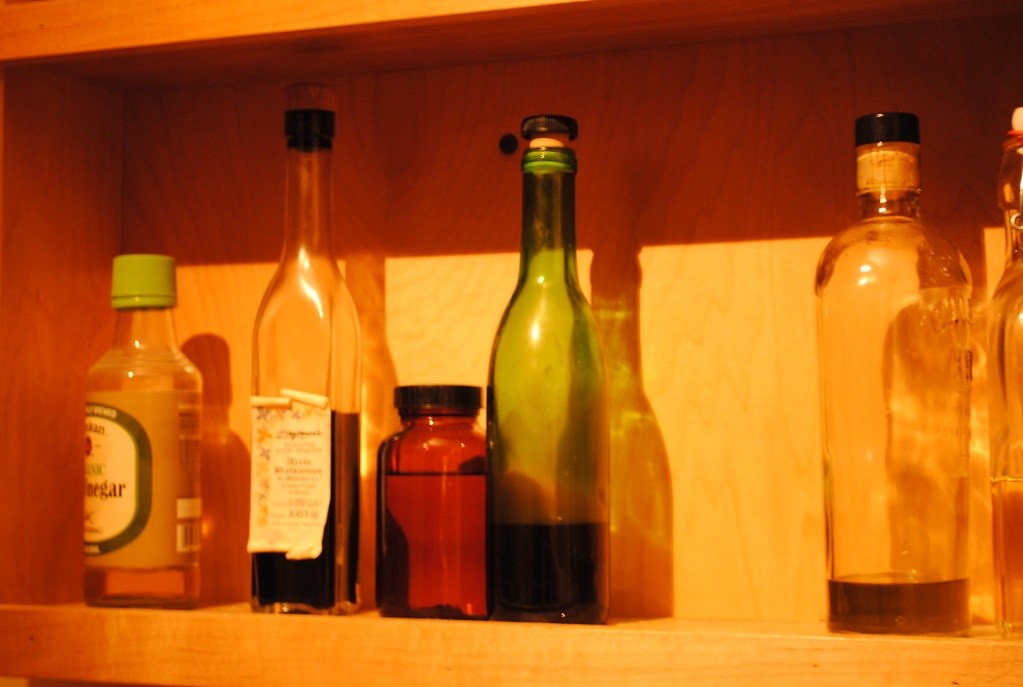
985 105 1023 641
245 83 364 617
81 252 203 610
374 386 487 617
814 110 975 638
484 113 613 626
